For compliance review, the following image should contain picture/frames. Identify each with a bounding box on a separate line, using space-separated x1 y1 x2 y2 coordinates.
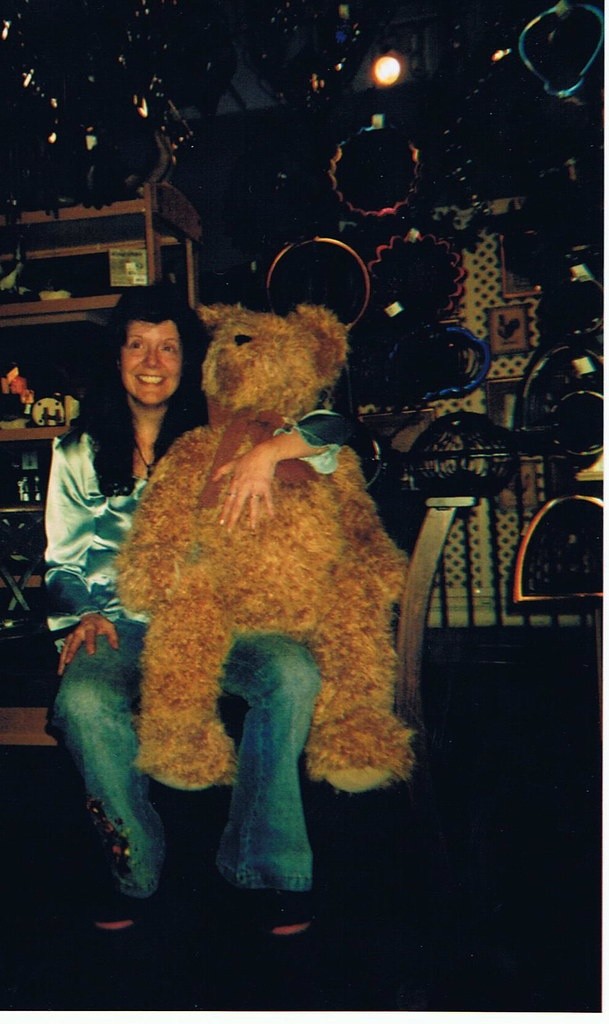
498 231 545 297
486 378 523 434
487 303 530 355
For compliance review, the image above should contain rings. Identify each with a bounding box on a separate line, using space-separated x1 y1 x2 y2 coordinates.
249 495 261 501
226 492 237 498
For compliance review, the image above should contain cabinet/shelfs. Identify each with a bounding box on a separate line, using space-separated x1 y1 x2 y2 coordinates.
0 184 206 636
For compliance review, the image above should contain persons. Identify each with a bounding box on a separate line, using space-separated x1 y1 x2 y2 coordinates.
37 278 353 950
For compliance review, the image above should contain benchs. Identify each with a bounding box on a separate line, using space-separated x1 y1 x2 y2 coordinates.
0 497 475 1012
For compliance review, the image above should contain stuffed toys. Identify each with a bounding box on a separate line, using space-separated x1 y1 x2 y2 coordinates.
109 303 419 795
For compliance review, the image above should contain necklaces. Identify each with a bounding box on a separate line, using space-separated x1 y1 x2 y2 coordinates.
132 440 157 477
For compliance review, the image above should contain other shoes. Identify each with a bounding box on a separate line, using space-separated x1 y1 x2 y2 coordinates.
260 891 315 948
89 897 141 937
